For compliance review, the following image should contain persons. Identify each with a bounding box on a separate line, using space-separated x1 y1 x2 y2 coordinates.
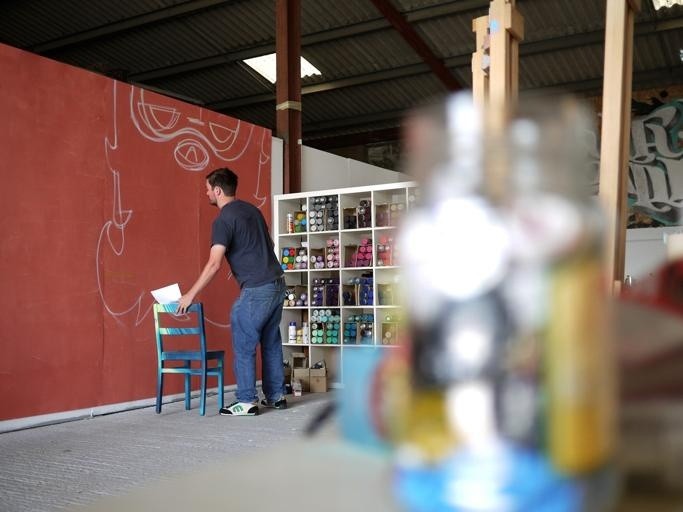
169 165 286 418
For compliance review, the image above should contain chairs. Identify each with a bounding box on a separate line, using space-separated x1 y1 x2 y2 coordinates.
153 304 224 416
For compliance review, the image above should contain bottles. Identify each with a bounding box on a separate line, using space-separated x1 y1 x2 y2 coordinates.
281 188 424 345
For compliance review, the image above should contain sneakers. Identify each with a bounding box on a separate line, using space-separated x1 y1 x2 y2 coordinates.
219 399 259 416
261 396 287 409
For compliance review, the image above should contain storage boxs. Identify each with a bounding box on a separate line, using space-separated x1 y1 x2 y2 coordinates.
282 352 329 393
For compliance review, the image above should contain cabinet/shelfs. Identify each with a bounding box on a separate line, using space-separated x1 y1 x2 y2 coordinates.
274 182 419 390
